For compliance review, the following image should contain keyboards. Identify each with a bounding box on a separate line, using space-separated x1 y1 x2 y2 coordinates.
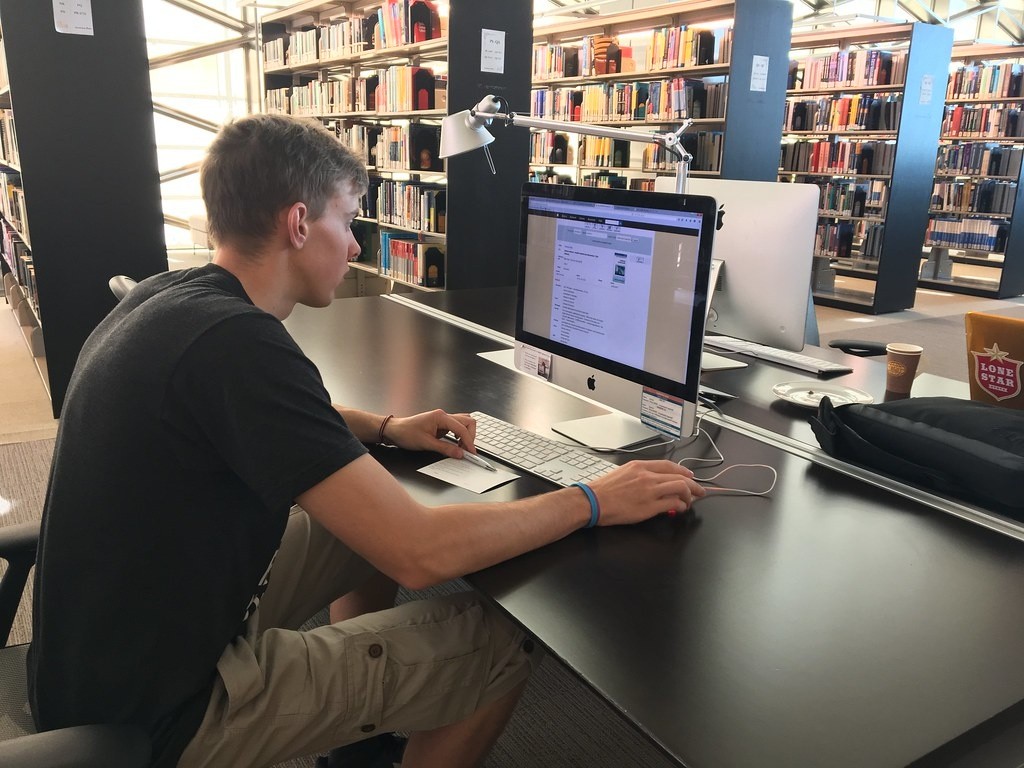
703 336 854 375
445 411 619 488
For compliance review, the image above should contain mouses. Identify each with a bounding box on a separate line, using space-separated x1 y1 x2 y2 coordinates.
662 493 680 514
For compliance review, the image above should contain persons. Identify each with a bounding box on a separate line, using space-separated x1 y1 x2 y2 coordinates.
25 117 706 768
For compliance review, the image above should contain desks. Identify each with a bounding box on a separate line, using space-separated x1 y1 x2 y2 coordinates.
139 283 1024 768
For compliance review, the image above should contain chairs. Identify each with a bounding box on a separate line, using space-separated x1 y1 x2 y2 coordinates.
0 502 135 768
805 284 889 363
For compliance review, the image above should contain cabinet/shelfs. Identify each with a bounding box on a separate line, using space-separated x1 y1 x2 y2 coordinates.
0 0 1024 420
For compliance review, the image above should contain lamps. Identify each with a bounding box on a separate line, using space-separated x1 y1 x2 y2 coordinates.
437 94 697 197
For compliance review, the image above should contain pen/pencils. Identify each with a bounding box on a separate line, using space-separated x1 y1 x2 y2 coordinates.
439 437 497 472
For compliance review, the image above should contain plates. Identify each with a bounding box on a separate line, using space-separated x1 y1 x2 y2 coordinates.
771 379 873 412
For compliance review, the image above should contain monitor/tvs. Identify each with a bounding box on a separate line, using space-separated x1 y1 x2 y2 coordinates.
654 177 819 371
514 182 717 452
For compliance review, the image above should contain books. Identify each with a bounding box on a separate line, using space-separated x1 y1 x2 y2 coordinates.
261 0 446 287
528 25 732 191
777 50 909 261
0 110 41 324
922 64 1024 252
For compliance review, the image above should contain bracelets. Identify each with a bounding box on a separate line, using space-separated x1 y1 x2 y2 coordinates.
376 414 393 446
568 483 600 529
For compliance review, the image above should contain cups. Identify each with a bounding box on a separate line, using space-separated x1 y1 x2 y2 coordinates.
885 343 924 395
